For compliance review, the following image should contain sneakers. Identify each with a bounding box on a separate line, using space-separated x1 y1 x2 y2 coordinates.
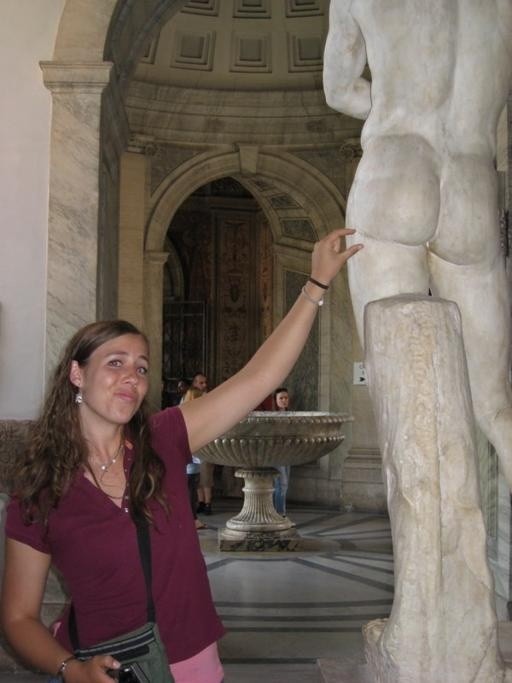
193 503 211 530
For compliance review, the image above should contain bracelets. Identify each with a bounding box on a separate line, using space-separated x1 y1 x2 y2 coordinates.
57 655 77 676
301 287 326 306
307 276 329 290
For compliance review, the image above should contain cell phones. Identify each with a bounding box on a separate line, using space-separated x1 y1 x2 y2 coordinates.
106 660 151 683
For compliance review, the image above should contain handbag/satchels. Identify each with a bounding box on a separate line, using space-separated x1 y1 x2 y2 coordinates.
72 621 175 682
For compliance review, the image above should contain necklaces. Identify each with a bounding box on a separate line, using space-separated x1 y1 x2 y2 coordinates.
77 443 125 471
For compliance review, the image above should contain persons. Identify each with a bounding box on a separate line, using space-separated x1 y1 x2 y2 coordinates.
179 387 209 529
272 388 291 520
0 228 361 683
321 1 512 500
178 381 190 393
161 376 173 410
178 376 217 514
